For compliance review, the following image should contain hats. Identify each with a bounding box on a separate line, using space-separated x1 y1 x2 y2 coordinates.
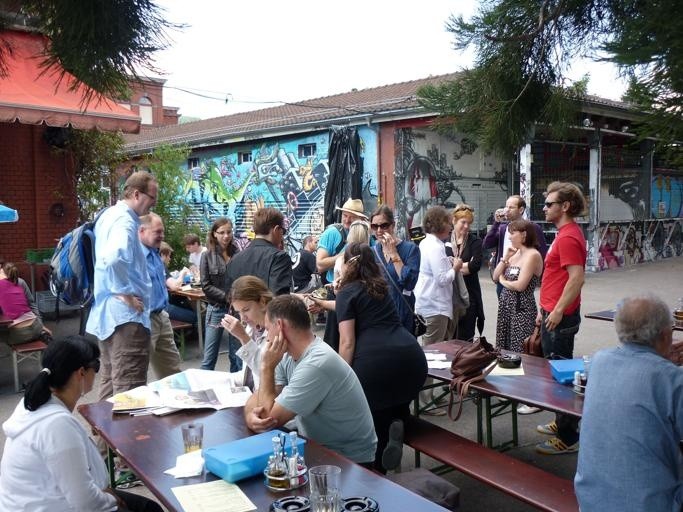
335 196 370 222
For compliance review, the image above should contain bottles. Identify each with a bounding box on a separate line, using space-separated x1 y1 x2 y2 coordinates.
266 431 305 487
574 354 590 390
673 297 683 324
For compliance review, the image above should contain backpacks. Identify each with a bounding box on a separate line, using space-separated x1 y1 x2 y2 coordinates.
48 219 100 309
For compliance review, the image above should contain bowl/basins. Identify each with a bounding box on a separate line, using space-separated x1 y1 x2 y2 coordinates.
590 265 601 273
498 353 522 368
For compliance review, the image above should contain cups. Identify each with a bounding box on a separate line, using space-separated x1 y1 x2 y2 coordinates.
308 464 342 512
181 423 203 453
496 208 510 219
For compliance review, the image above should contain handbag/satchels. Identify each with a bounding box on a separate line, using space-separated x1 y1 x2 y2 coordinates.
449 336 498 375
522 321 546 359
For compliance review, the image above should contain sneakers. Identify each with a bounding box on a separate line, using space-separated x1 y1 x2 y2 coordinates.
516 404 544 414
535 433 581 454
537 419 558 436
381 418 405 471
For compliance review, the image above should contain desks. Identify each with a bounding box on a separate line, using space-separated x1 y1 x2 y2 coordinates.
1 217 227 393
0 305 682 512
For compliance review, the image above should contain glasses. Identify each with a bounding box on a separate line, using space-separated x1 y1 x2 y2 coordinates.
370 223 390 230
544 202 564 208
82 359 100 373
278 225 286 236
453 206 473 213
